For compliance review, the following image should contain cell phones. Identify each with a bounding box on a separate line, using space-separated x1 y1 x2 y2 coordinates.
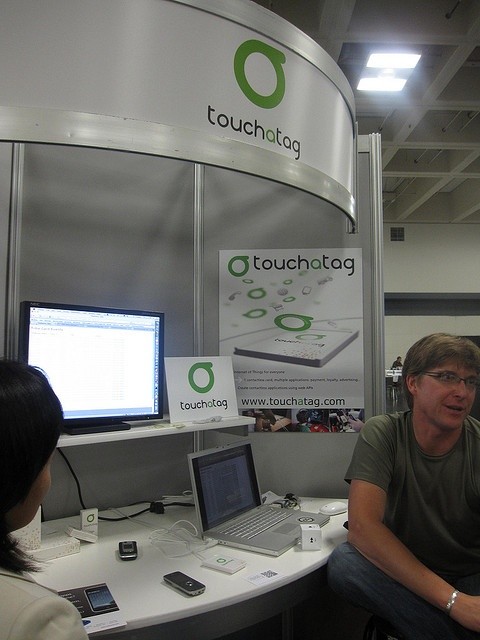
163 571 206 596
119 541 137 561
349 414 355 421
84 585 118 614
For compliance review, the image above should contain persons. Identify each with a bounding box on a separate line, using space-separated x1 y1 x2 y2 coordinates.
0 360 89 640
348 418 365 431
294 408 312 432
390 356 402 370
327 334 480 639
310 410 329 432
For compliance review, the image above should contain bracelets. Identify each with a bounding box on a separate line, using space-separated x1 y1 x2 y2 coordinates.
444 588 459 617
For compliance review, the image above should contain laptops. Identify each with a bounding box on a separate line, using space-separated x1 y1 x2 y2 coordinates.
187 439 330 557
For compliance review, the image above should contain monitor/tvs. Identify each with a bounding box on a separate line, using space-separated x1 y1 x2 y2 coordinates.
18 300 164 435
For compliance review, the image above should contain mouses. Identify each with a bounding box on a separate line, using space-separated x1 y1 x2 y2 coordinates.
319 501 348 515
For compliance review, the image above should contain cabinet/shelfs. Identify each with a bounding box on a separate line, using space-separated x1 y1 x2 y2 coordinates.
48 408 258 454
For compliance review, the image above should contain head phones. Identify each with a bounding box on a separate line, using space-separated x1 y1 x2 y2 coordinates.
274 493 301 509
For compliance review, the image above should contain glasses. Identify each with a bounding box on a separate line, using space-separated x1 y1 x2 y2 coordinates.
419 370 480 389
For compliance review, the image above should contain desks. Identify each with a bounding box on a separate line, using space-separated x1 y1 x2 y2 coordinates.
10 490 350 640
